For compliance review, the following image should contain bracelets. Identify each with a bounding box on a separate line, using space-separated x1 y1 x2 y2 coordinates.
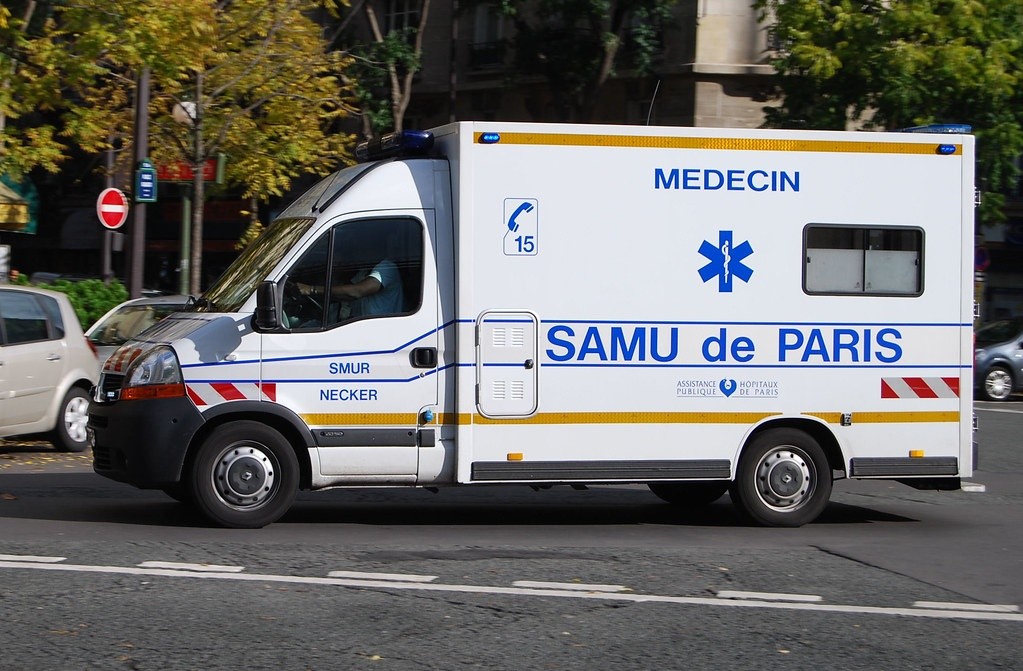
310 283 317 295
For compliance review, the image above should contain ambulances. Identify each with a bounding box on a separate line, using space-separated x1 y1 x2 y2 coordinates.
85 119 975 529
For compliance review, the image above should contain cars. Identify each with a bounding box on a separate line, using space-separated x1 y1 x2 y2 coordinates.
975 317 1023 401
0 283 201 453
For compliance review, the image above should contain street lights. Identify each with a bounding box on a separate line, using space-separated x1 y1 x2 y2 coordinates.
170 101 197 296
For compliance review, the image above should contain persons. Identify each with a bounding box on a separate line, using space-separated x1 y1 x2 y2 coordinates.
0 269 19 285
290 234 403 328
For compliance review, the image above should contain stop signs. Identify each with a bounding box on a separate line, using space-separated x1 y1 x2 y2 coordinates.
97 187 130 229
